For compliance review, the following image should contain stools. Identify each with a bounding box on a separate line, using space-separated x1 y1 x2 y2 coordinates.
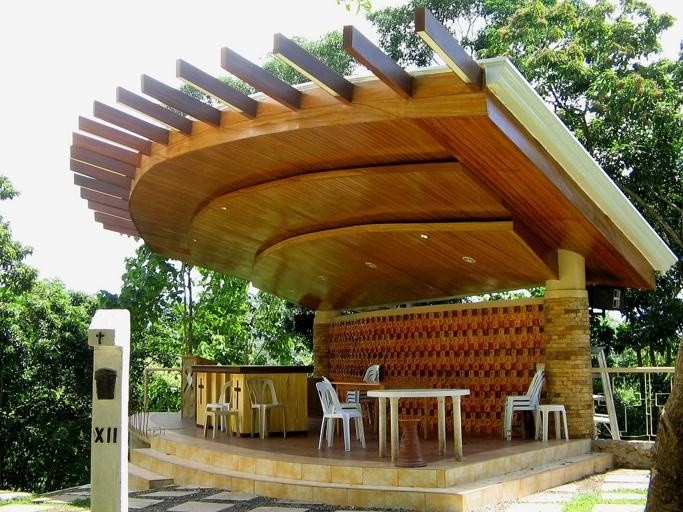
534 404 569 443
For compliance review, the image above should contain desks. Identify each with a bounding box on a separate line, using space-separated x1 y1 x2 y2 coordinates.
365 387 471 465
329 380 387 441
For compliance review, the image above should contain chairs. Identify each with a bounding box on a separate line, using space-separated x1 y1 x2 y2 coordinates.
502 368 548 442
203 378 288 442
315 363 383 453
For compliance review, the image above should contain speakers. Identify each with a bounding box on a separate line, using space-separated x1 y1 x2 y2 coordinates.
298 314 315 336
586 284 625 310
